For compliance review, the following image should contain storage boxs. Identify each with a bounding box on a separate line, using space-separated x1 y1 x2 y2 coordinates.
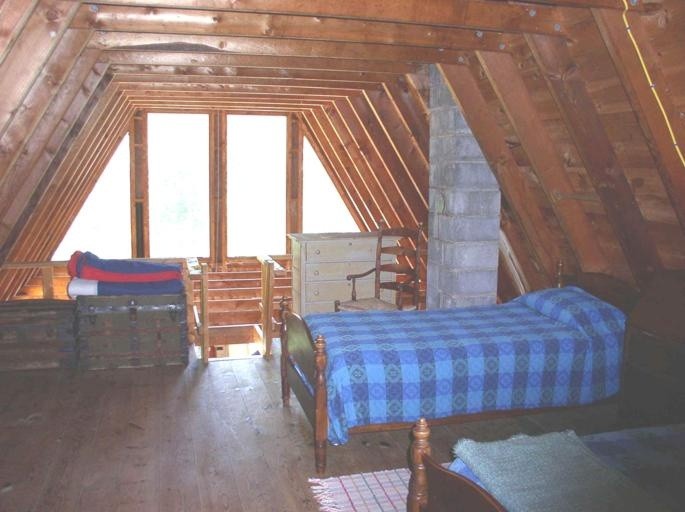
74 293 190 367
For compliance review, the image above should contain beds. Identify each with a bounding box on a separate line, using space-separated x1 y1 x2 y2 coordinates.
280 260 638 472
404 418 684 512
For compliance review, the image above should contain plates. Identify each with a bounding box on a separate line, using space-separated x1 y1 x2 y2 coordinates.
286 232 403 318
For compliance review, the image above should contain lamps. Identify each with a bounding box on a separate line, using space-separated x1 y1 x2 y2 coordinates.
335 218 425 312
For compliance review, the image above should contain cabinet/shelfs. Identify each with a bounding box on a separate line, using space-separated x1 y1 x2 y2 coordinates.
286 232 403 318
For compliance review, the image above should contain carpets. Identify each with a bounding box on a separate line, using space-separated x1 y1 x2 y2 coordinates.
307 461 450 509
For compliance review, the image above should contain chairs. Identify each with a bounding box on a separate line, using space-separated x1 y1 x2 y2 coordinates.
335 218 425 312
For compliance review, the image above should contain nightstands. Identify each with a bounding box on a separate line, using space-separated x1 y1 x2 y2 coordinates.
621 271 684 426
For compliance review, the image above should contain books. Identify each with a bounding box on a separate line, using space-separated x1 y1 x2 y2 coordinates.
74 293 190 367
